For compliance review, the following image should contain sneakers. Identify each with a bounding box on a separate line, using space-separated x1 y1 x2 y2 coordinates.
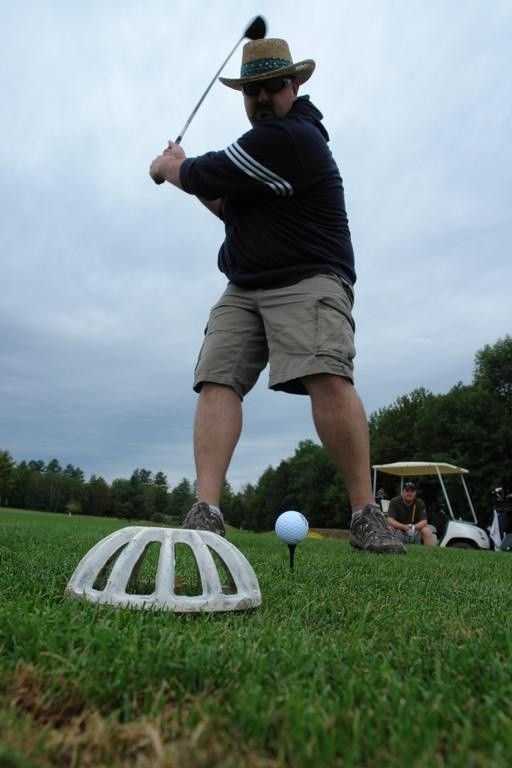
179 502 227 537
347 503 406 555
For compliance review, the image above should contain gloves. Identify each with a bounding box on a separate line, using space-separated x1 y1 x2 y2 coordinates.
406 523 416 536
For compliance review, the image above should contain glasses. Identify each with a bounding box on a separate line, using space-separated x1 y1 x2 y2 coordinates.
239 74 295 98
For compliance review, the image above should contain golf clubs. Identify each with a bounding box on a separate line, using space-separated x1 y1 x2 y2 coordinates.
155 17 266 185
491 486 512 506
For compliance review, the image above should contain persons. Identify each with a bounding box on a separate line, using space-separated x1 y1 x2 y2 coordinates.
146 35 407 555
386 481 434 545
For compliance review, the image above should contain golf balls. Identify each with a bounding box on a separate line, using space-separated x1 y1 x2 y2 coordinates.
275 510 309 543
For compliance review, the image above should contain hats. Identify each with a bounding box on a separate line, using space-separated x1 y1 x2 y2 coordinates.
403 481 417 491
218 37 316 90
